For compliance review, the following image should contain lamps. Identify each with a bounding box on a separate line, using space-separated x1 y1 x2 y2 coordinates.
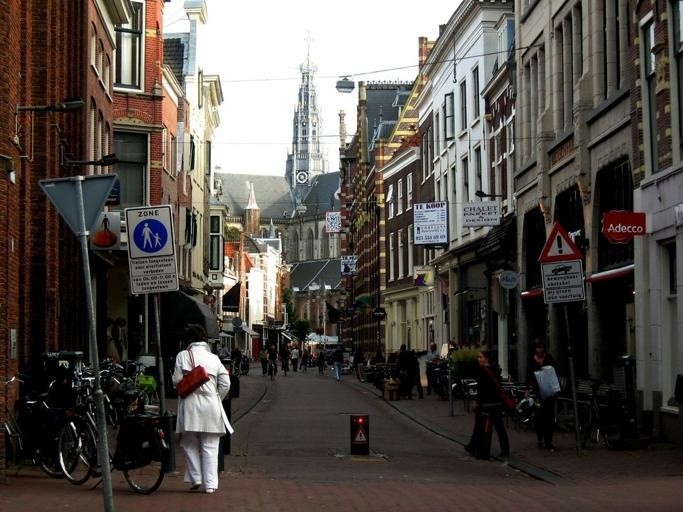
66 152 119 167
18 97 84 113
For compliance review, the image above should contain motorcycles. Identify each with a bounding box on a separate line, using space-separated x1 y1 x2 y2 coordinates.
424 356 450 400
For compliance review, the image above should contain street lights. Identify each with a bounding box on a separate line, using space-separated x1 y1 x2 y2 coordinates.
315 295 327 350
280 303 288 330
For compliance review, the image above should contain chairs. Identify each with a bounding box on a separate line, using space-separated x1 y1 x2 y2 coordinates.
554 376 610 428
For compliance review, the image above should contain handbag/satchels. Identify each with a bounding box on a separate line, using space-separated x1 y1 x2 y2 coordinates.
176 364 210 400
113 415 173 471
515 397 541 424
501 390 516 415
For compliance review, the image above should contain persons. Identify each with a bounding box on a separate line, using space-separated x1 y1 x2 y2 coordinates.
170 324 234 493
464 346 510 461
523 342 563 450
218 339 483 401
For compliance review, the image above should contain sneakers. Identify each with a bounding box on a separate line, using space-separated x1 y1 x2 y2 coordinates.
206 488 214 493
536 440 556 451
190 480 203 491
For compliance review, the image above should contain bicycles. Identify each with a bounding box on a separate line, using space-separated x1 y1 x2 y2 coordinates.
267 359 277 379
0 348 172 493
279 358 290 376
